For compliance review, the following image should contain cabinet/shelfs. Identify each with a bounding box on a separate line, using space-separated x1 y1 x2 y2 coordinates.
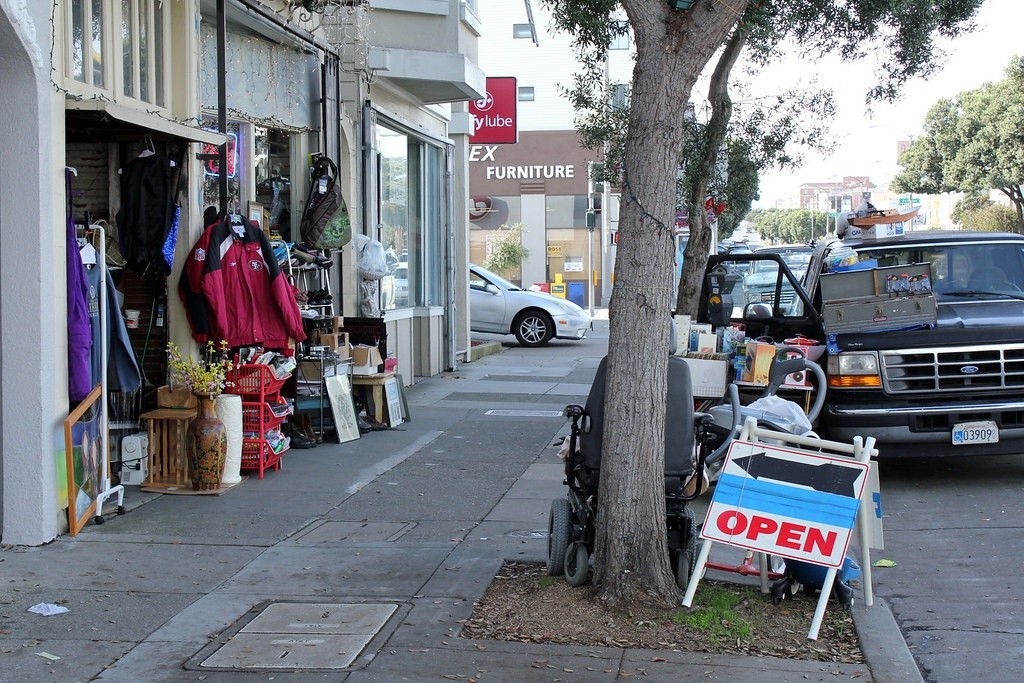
268 236 335 315
225 353 292 480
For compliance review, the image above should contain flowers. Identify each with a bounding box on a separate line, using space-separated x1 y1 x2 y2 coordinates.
165 339 236 394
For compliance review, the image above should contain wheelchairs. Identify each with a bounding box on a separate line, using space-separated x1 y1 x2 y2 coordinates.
543 314 702 593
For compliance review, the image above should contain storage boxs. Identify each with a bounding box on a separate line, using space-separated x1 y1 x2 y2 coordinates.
733 339 809 387
673 352 731 398
844 223 862 239
346 344 383 375
817 262 938 338
847 214 919 239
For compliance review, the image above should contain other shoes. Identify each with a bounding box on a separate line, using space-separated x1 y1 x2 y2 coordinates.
363 415 388 430
356 416 372 433
283 427 324 447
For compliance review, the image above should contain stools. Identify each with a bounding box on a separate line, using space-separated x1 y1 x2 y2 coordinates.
135 407 198 490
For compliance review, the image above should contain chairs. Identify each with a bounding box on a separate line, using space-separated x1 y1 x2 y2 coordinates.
964 267 1009 293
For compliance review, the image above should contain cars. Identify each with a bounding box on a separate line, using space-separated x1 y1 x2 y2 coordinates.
388 246 591 351
695 199 1024 473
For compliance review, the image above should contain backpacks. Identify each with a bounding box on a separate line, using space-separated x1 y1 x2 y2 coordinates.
302 157 352 249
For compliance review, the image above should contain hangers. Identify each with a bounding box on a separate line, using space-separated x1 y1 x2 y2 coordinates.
226 195 247 235
79 224 98 264
143 133 156 156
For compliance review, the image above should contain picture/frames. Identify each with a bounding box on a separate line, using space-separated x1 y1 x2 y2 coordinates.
323 373 361 444
63 384 110 537
384 377 404 427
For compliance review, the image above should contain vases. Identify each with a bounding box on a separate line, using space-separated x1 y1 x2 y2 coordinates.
214 394 244 484
187 393 227 492
